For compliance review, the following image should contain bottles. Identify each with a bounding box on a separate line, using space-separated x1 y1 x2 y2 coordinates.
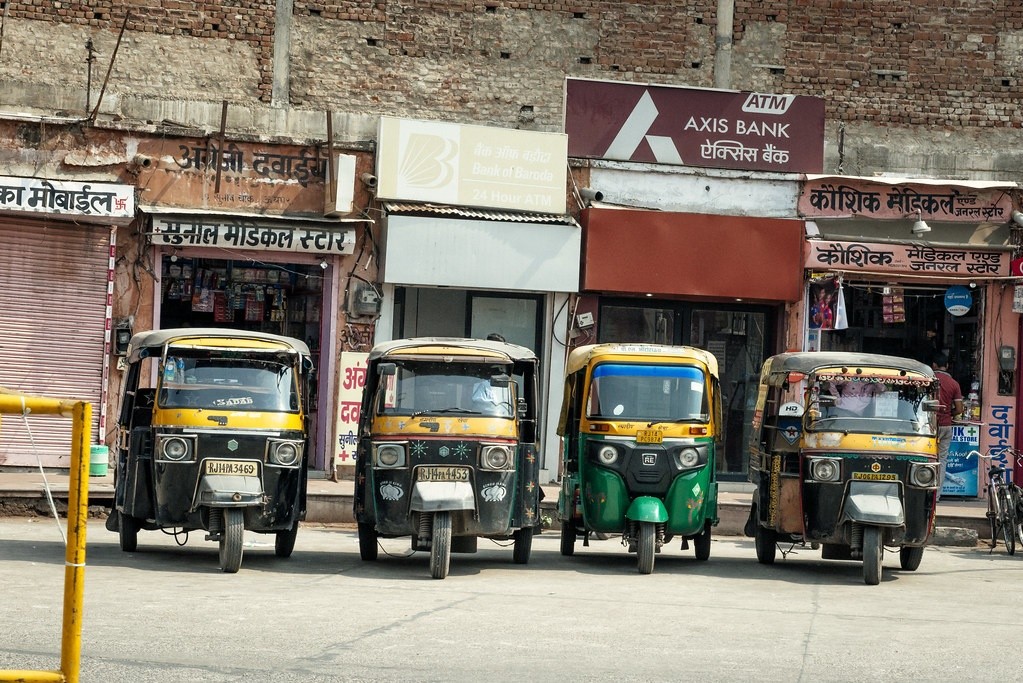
165 356 184 384
961 397 981 421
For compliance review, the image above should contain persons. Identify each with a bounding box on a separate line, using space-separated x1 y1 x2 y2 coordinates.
824 376 886 418
927 350 963 503
487 333 505 342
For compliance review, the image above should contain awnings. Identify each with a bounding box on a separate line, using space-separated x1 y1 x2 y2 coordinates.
383 201 580 227
139 205 375 224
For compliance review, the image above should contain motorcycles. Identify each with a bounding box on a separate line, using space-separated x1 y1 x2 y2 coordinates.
555 342 724 577
106 325 316 574
742 348 948 586
351 336 544 582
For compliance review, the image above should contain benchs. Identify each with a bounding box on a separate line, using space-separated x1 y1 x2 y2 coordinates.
130 388 157 455
769 414 803 477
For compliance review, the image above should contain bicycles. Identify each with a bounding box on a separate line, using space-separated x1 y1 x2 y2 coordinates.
965 443 1023 556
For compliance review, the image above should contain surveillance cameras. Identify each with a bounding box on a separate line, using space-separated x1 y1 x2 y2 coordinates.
912 220 931 234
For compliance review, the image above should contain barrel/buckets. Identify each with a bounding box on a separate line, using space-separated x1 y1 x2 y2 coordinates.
90 445 109 475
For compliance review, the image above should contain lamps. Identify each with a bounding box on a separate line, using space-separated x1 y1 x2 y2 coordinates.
904 209 932 237
